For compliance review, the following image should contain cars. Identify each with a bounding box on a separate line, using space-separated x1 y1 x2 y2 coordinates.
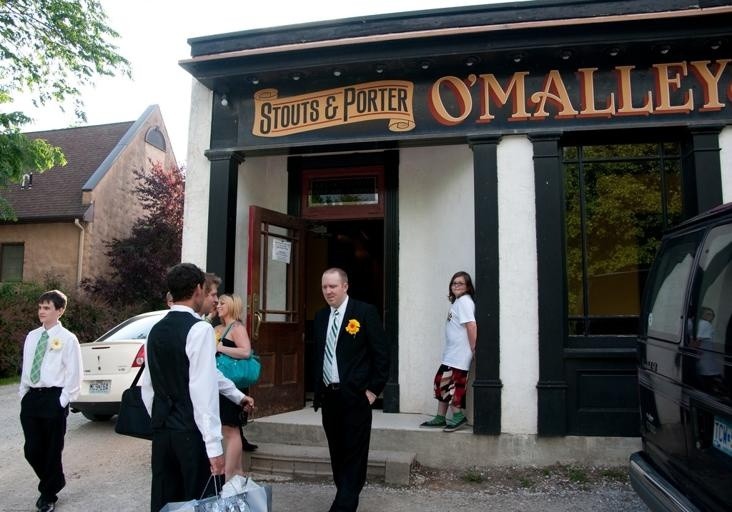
69 310 171 422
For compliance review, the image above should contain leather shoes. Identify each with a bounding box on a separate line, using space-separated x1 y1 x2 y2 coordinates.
242 438 258 450
38 499 58 512
36 482 66 508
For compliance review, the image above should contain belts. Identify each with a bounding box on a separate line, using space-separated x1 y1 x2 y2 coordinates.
322 383 341 390
29 388 55 392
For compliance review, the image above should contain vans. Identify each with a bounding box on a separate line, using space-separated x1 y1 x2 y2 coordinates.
626 202 730 511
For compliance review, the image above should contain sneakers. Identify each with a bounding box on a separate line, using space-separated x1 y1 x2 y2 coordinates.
444 416 468 432
420 420 447 427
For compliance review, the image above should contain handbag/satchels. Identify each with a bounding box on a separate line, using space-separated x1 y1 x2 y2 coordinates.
158 472 272 511
114 385 154 440
215 349 261 389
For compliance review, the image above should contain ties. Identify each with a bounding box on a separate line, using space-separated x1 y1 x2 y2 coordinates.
30 331 50 384
323 310 340 388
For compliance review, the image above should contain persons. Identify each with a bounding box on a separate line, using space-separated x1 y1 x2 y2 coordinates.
19 290 83 512
141 262 258 512
314 266 391 512
422 272 477 431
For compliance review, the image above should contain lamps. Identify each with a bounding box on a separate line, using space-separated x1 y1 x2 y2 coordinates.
250 39 719 87
220 90 229 107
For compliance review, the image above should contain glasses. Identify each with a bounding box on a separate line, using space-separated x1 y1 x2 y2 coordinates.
451 281 465 288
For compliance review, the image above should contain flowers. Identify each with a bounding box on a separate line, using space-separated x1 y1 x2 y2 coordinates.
48 339 60 353
344 319 361 338
